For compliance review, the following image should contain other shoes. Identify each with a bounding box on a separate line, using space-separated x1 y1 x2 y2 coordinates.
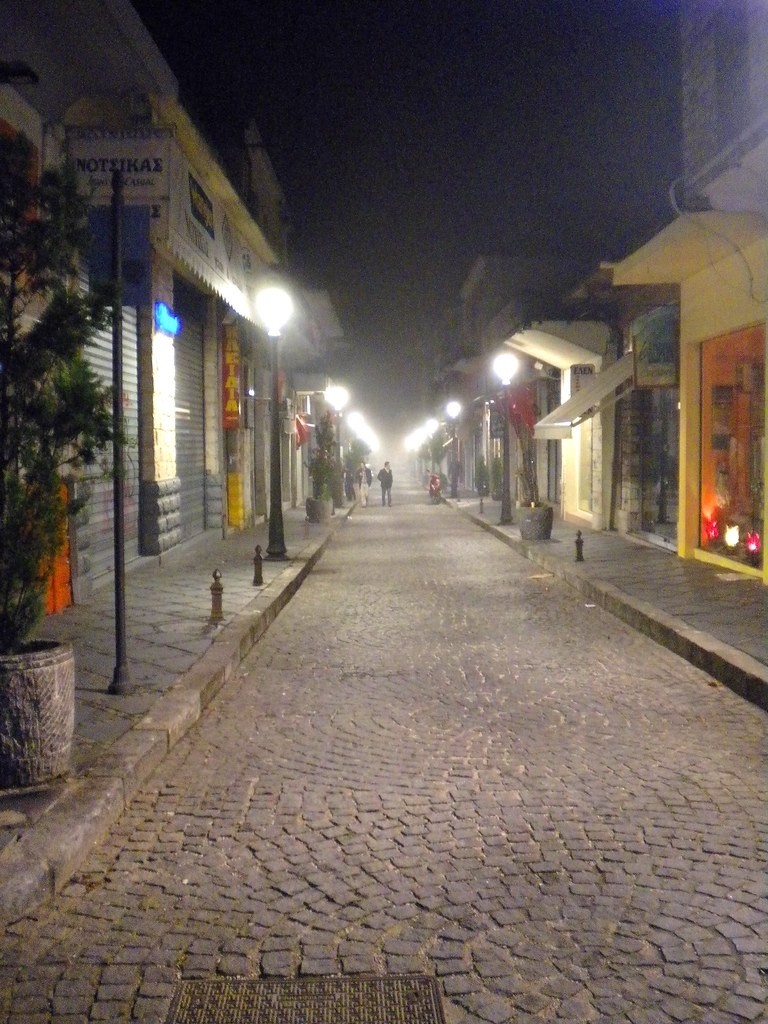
382 504 392 507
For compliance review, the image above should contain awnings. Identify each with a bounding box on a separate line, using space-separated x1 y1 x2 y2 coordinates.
532 353 635 440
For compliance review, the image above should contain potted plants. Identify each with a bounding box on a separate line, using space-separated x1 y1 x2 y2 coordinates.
0 126 132 789
492 454 503 502
516 417 553 541
304 410 340 523
475 456 490 496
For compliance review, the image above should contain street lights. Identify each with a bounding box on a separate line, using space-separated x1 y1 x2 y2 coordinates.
254 285 292 562
446 399 462 499
491 353 519 526
327 385 350 462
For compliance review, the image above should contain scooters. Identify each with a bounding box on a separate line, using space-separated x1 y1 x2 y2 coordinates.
425 468 443 505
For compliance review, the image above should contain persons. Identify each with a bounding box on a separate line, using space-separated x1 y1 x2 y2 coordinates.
377 461 393 507
356 462 372 508
